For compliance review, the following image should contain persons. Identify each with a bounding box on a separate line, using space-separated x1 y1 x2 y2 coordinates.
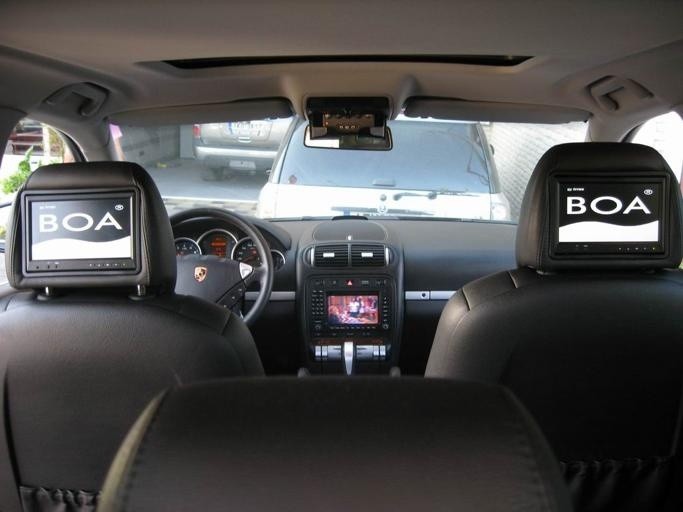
328 295 377 326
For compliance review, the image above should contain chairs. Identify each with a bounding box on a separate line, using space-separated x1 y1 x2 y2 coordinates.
423 140 683 512
0 158 265 512
95 375 577 512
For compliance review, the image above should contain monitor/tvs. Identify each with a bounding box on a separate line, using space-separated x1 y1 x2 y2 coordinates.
26 193 134 272
554 177 665 255
327 294 379 326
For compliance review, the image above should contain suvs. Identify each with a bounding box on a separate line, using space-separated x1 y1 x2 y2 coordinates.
256 115 510 228
191 116 293 181
